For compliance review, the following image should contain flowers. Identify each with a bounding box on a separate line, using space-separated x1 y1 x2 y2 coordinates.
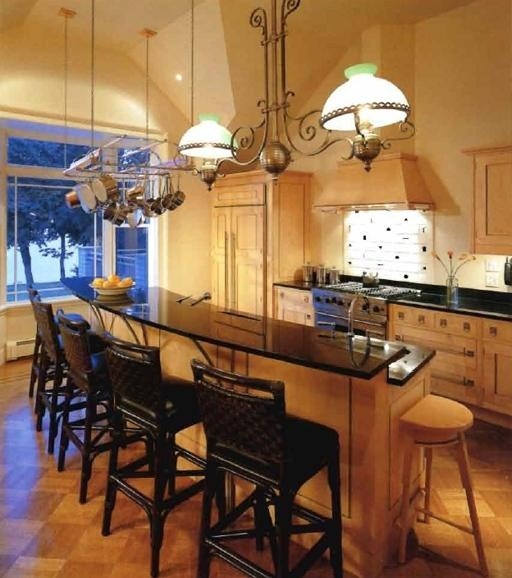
430 248 477 301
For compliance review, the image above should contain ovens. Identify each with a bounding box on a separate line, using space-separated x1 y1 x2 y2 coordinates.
312 307 388 341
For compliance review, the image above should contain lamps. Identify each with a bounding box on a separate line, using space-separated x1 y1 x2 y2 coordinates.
178 0 417 191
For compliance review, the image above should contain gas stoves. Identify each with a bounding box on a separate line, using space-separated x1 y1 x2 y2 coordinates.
316 281 421 300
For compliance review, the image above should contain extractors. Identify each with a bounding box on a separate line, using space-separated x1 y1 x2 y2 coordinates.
311 24 433 214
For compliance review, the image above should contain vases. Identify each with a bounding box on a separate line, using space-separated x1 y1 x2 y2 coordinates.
446 276 459 305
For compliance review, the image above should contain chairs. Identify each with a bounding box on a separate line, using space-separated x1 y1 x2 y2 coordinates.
191 358 343 578
26 283 84 416
30 295 113 454
102 336 226 577
56 308 155 504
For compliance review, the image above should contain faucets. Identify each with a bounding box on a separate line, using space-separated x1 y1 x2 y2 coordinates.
347 293 367 337
348 335 370 368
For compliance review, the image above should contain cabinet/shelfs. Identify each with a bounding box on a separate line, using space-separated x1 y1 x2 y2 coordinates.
210 169 314 319
274 284 315 327
462 144 511 257
483 318 511 428
387 303 481 421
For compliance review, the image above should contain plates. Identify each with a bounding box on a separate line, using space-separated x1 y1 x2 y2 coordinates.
88 282 136 296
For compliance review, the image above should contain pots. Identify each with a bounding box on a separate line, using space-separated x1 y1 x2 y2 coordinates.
63 175 185 228
301 261 314 282
315 264 327 284
330 267 341 285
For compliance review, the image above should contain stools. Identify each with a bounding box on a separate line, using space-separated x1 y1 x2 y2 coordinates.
397 394 489 578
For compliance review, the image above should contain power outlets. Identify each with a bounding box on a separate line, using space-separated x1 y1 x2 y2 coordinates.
485 258 500 272
485 272 500 288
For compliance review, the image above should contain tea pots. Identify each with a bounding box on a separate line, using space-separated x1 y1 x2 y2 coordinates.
363 272 380 289
